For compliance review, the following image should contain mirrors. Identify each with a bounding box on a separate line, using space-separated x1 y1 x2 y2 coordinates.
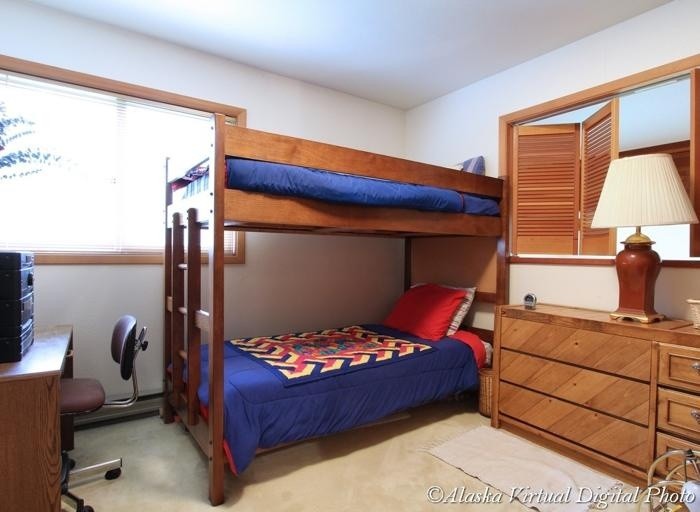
500 55 700 270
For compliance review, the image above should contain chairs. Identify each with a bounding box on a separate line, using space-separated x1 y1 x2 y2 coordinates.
60 314 149 512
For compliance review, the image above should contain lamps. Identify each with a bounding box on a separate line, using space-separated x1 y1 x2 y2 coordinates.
590 152 700 324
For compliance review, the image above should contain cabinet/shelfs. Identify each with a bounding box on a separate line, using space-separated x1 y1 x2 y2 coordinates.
489 298 700 503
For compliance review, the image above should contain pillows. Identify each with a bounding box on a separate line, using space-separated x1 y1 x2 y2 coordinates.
377 278 478 342
456 154 485 176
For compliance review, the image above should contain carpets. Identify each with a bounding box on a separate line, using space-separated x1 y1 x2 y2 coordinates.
430 423 620 512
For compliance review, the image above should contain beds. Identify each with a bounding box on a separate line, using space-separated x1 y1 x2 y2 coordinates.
158 111 509 508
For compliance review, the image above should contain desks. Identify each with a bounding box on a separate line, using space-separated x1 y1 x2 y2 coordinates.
0 329 74 512
